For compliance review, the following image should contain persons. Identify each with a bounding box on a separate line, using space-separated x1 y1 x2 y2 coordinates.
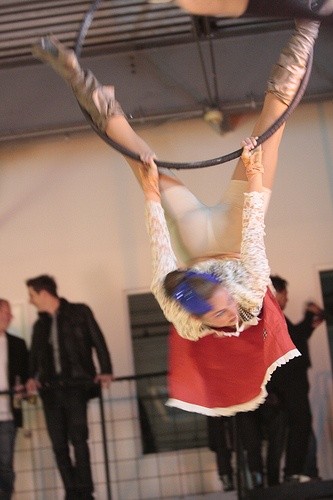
32 0 333 417
208 276 324 492
0 300 29 500
26 275 113 500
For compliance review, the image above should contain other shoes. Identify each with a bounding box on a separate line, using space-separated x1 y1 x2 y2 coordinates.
222 475 233 490
